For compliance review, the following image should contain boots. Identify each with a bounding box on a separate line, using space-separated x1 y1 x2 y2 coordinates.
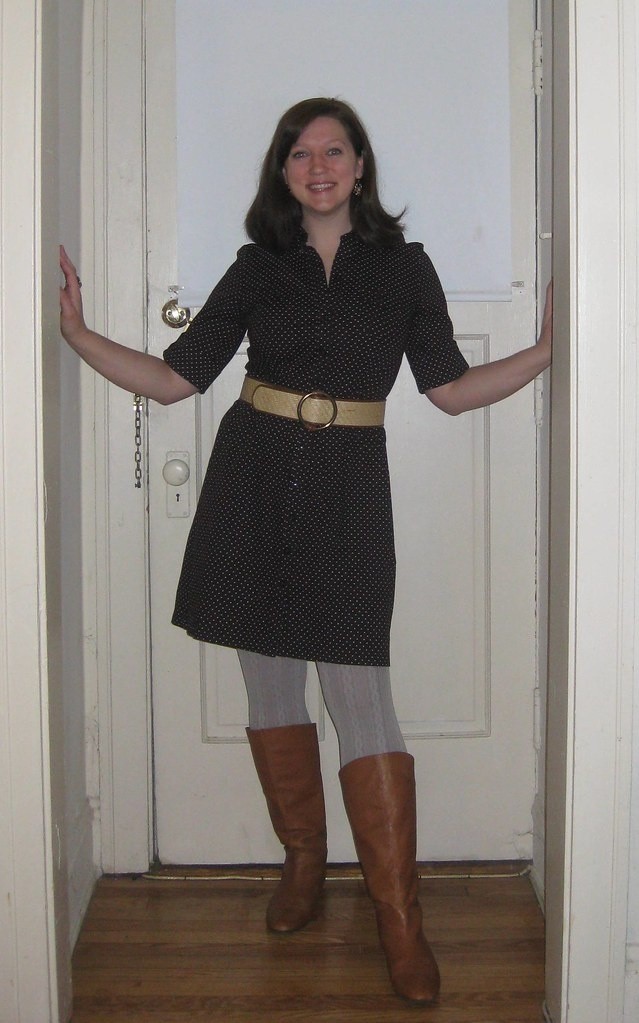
244 723 328 934
337 751 441 1002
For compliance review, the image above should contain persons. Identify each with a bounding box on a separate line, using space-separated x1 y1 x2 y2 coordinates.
58 96 554 1005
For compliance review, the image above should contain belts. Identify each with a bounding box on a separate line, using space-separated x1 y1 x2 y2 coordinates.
239 376 387 431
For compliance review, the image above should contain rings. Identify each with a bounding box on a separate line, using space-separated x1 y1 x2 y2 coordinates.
76 276 82 288
